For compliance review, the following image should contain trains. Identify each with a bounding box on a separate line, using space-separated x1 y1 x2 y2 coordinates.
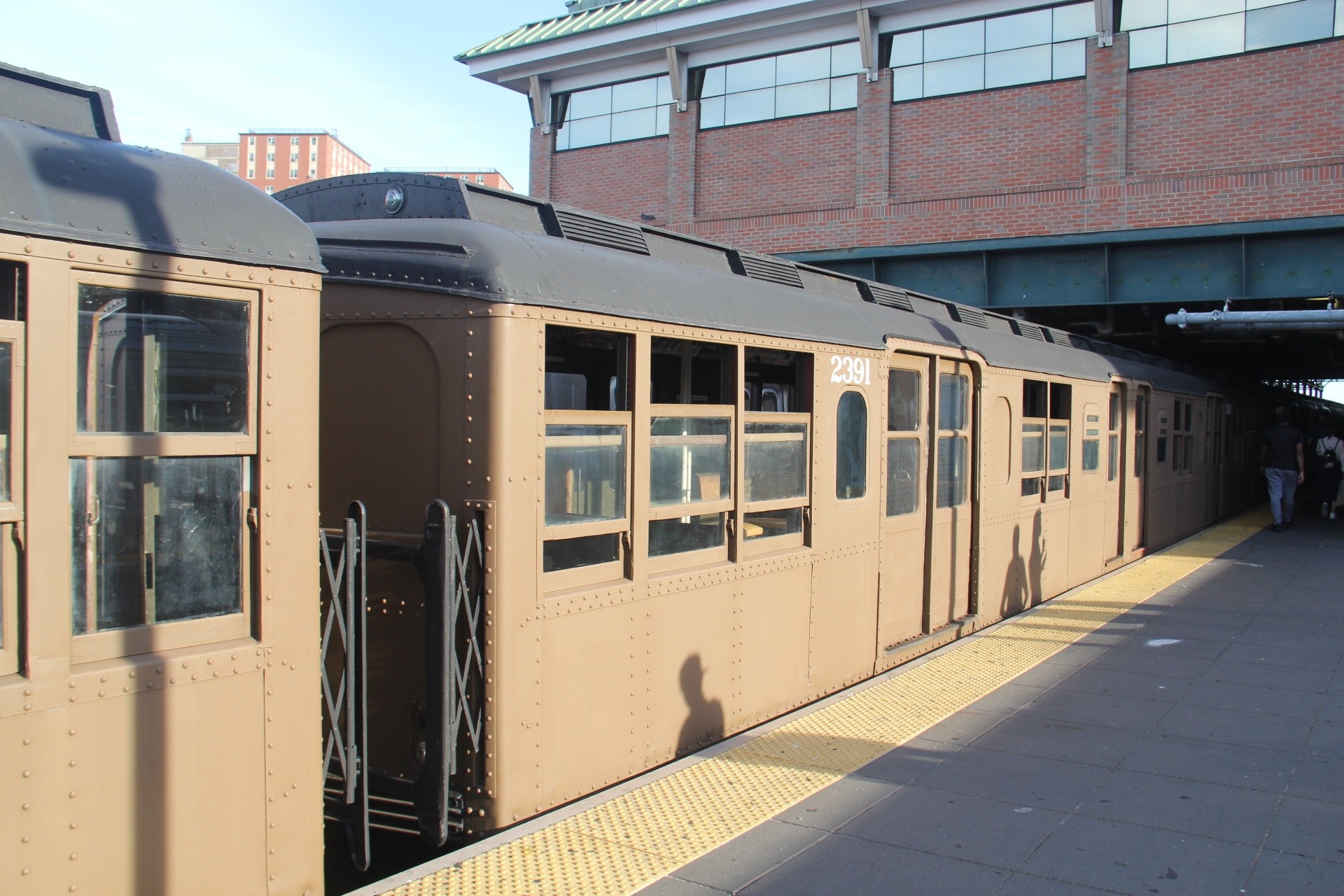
0 57 1343 895
